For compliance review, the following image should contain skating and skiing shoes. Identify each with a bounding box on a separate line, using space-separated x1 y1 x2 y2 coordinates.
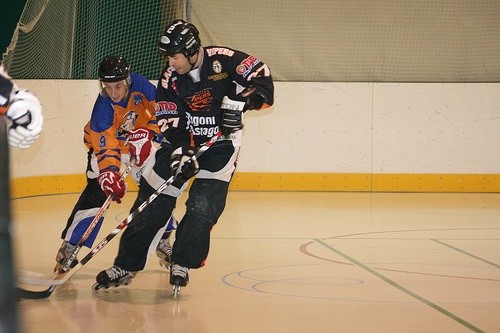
170 263 189 299
156 240 173 269
54 242 81 274
91 266 137 293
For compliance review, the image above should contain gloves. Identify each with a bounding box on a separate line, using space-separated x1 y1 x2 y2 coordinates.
128 127 156 166
169 147 199 181
222 110 245 134
97 170 126 204
8 89 43 149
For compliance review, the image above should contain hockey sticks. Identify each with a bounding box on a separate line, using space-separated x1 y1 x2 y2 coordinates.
15 132 223 286
13 154 136 299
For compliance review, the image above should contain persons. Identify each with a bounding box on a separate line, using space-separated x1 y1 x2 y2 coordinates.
0 63 43 149
53 55 178 275
92 18 275 298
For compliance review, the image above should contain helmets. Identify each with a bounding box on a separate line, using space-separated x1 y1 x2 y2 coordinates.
97 55 129 82
158 19 201 56
